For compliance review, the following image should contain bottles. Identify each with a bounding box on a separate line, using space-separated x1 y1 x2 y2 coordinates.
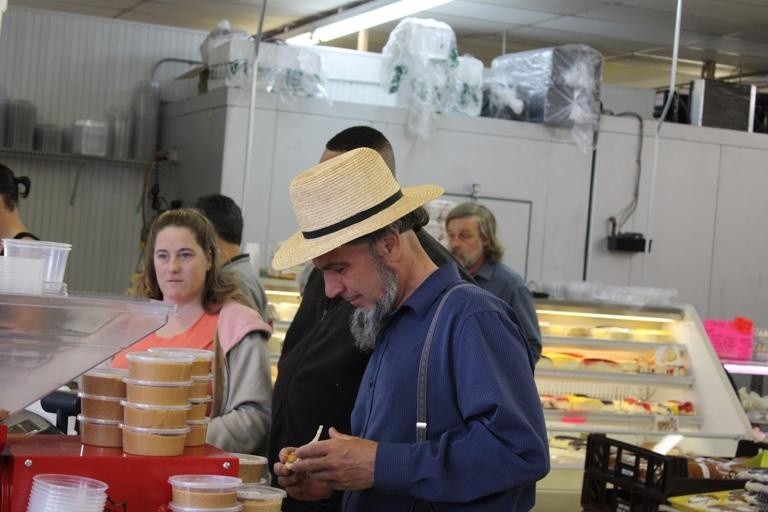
72 116 110 157
2 97 63 155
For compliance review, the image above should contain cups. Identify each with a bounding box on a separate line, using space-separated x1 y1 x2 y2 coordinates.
111 79 161 161
244 242 259 280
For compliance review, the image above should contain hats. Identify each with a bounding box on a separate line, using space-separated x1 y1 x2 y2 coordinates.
271 147 444 272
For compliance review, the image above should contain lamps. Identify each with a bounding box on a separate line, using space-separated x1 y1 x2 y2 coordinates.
261 0 461 48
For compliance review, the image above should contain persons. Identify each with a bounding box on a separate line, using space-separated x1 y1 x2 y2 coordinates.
269 126 482 512
103 207 275 452
268 147 550 511
194 192 270 323
444 204 542 376
0 163 45 259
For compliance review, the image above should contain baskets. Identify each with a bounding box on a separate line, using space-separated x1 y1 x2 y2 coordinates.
580 431 767 512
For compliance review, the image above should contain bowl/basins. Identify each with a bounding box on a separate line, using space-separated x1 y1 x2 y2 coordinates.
78 345 217 456
23 472 108 512
1 237 74 295
168 451 289 512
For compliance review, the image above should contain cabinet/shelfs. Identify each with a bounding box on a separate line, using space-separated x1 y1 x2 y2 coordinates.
247 277 754 512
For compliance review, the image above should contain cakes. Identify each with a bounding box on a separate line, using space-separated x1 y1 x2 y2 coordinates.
538 391 702 416
687 467 768 512
547 432 685 466
608 446 747 482
535 345 690 380
536 324 675 343
